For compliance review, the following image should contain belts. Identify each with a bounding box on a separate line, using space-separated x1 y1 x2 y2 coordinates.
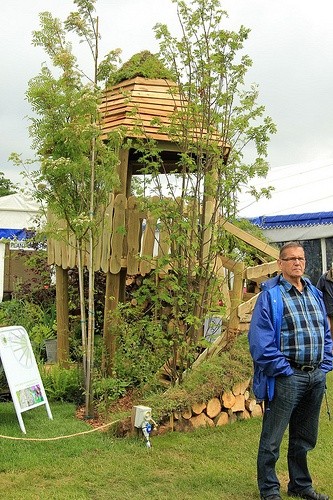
290 365 318 373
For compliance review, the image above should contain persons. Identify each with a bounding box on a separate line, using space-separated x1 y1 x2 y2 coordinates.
248 243 333 500
316 261 333 355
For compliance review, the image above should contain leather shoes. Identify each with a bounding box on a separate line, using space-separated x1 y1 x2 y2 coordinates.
261 495 282 500
288 485 328 500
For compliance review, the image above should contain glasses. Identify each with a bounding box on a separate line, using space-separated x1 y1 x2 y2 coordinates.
282 257 305 261
